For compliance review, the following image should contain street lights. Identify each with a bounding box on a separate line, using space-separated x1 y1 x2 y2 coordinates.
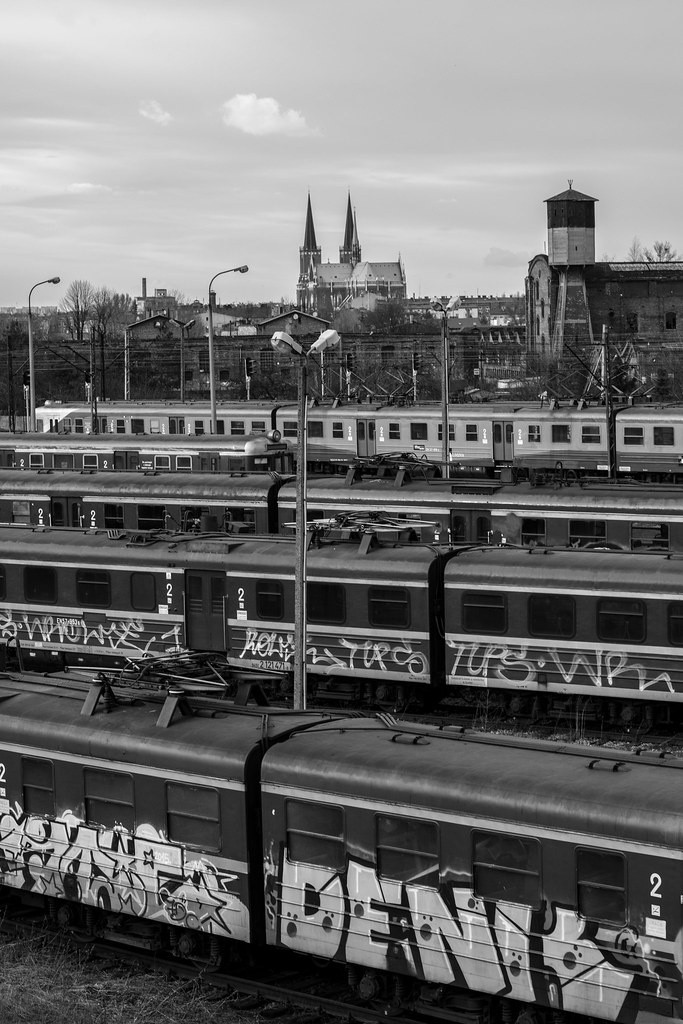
428 289 468 478
271 328 344 714
204 263 250 436
25 275 61 434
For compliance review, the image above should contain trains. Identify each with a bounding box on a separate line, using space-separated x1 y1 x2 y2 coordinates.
0 521 681 747
29 397 681 473
0 467 681 560
0 663 682 1024
